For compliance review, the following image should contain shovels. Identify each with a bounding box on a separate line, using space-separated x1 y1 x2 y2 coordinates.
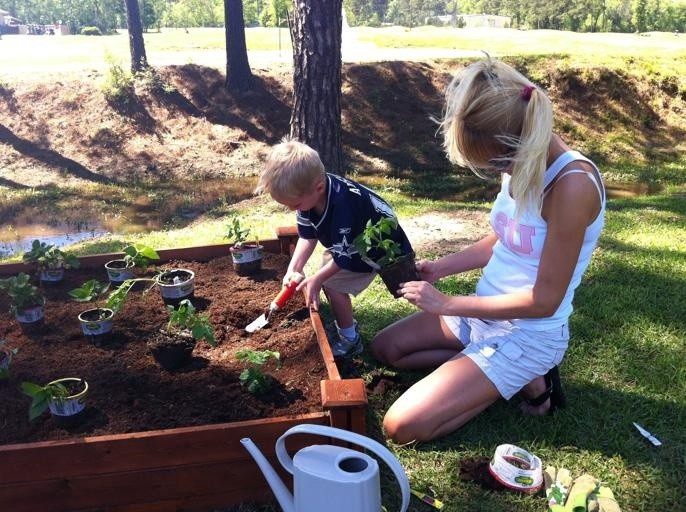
244 272 304 333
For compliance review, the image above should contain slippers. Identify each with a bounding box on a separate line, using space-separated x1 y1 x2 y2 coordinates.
521 365 566 411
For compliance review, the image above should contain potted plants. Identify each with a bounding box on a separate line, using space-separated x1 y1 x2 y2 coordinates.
348 215 419 298
18 378 89 426
146 267 194 310
24 239 80 287
104 243 159 287
68 278 132 344
0 272 45 333
224 213 269 276
0 337 16 379
148 304 214 369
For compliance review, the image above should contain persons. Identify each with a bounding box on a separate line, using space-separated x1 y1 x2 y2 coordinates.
370 49 607 450
254 132 416 358
26 23 46 35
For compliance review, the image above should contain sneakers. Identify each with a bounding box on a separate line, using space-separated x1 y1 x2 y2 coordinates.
331 334 363 358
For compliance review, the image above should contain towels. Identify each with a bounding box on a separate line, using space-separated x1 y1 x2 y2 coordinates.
545 466 621 511
